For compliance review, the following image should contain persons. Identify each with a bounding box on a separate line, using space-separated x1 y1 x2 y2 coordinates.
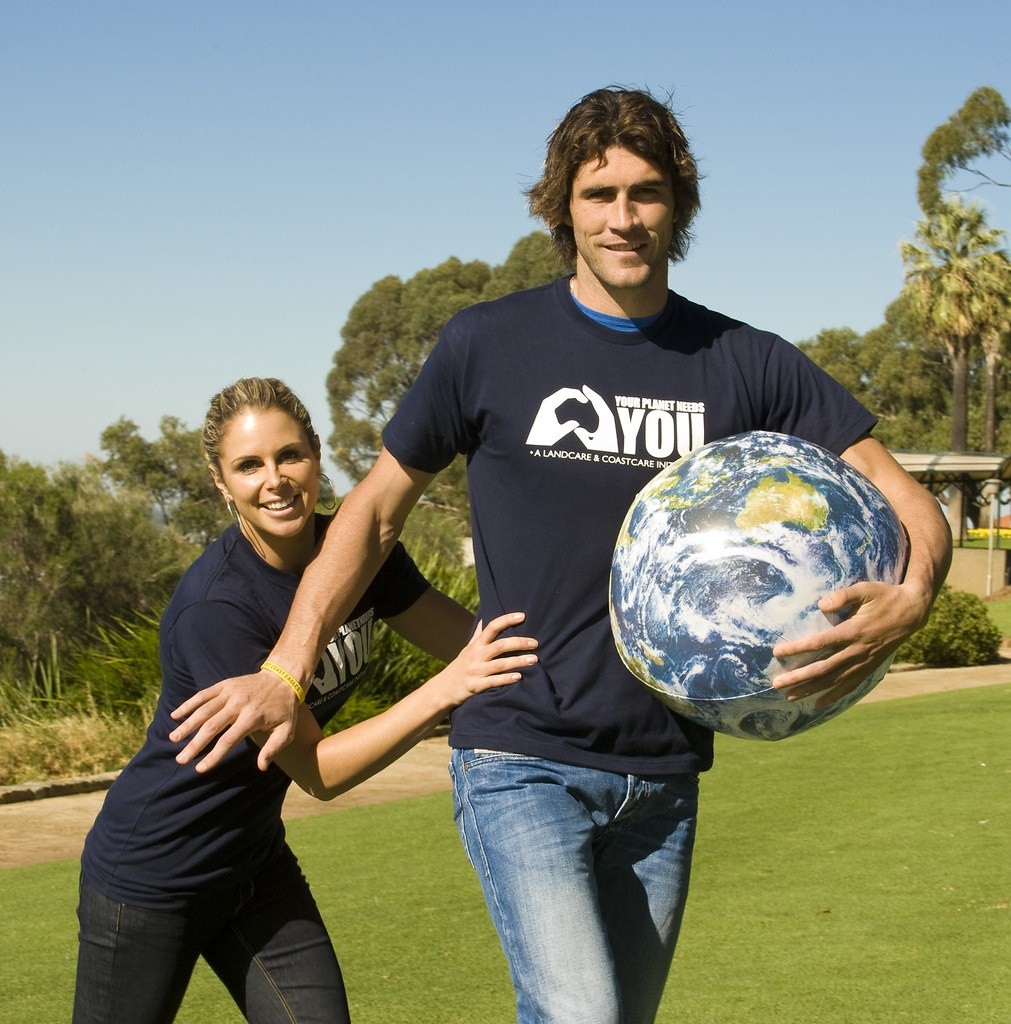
74 376 539 1022
169 87 952 1024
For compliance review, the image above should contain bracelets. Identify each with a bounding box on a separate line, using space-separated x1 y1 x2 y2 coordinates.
260 661 305 705
